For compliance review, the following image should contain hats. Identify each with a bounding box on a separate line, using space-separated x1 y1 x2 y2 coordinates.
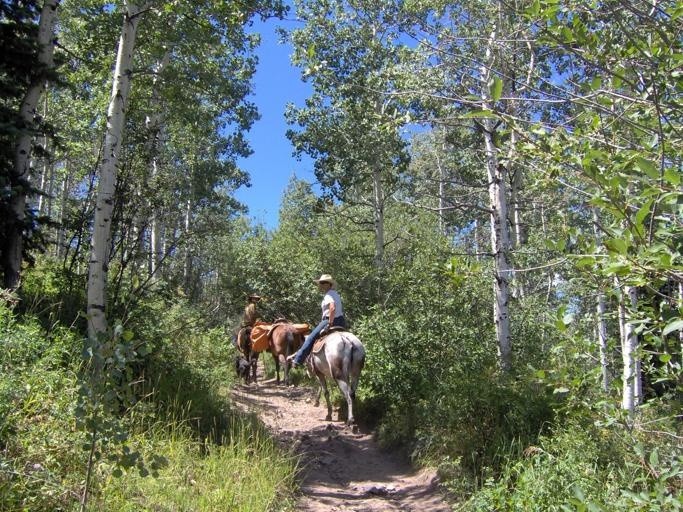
313 274 337 285
247 292 261 300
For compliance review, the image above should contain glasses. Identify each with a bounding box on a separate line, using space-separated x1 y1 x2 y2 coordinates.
318 282 327 285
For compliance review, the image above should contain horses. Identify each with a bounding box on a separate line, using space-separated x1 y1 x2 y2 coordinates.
268 322 304 388
237 327 261 383
304 330 366 426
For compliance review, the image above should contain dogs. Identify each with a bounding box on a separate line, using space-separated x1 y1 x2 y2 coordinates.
236 356 257 384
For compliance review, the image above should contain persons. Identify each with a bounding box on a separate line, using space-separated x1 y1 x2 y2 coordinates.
239 293 265 330
286 273 346 369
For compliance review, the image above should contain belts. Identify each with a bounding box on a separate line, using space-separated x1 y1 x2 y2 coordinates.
325 317 329 319
